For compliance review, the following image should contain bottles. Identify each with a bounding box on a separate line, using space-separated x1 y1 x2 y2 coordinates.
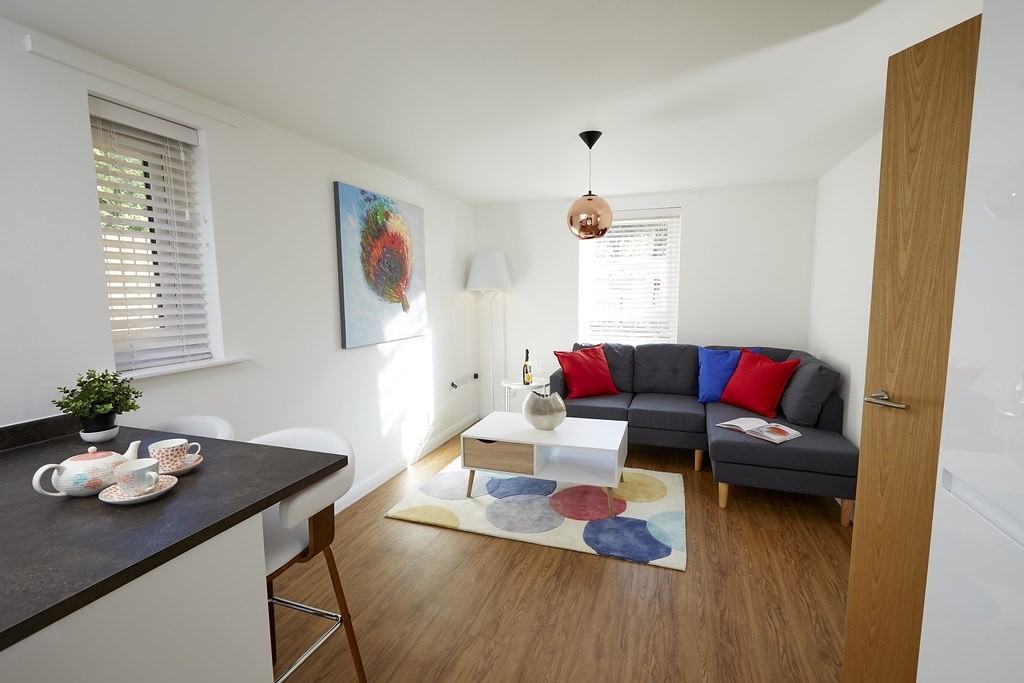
523 349 532 385
523 390 566 430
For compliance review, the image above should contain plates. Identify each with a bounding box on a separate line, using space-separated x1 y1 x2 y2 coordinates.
98 475 178 504
159 453 204 476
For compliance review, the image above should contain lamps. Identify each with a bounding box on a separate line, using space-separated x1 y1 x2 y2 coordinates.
466 250 512 444
567 131 612 239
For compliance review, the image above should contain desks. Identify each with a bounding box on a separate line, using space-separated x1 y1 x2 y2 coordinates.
0 414 348 683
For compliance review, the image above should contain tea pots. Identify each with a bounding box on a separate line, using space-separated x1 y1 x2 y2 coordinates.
32 440 142 496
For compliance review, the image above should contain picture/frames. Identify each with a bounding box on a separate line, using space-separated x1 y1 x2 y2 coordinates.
334 182 424 349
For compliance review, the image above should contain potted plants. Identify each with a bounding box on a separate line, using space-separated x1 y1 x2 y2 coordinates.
54 367 139 441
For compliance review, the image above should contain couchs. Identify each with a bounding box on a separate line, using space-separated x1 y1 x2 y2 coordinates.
550 342 860 526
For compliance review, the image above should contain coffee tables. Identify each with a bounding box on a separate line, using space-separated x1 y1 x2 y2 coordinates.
462 411 628 518
503 378 548 411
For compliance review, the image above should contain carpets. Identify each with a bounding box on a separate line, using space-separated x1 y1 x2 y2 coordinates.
385 455 686 571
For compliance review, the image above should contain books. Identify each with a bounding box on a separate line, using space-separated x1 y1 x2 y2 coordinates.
716 418 802 444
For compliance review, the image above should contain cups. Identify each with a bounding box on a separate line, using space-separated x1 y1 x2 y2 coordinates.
115 458 159 496
148 438 201 471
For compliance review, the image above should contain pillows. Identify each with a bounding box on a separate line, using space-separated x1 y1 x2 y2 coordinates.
554 345 620 399
699 347 800 417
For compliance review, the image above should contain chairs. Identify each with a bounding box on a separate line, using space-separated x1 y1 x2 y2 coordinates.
140 416 367 683
